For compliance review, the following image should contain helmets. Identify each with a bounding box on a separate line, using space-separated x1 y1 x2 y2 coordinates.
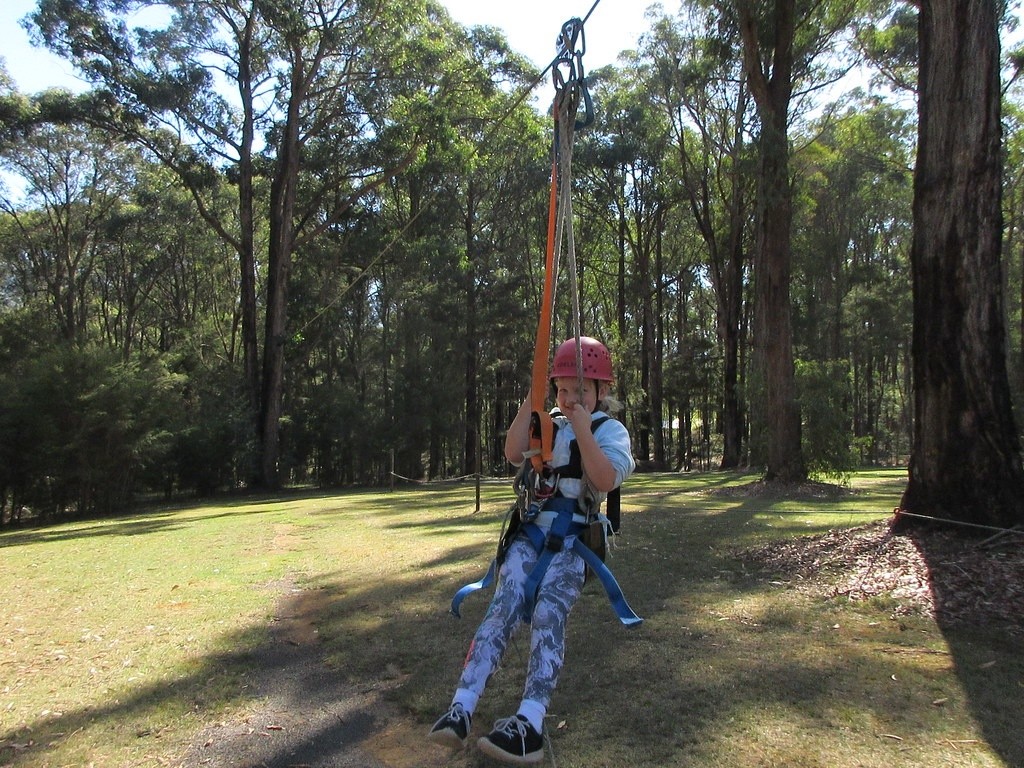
550 336 619 381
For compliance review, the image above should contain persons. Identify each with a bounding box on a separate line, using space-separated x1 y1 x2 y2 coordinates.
428 335 635 764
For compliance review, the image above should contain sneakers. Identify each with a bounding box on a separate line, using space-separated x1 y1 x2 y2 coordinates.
431 703 473 748
478 715 546 762
